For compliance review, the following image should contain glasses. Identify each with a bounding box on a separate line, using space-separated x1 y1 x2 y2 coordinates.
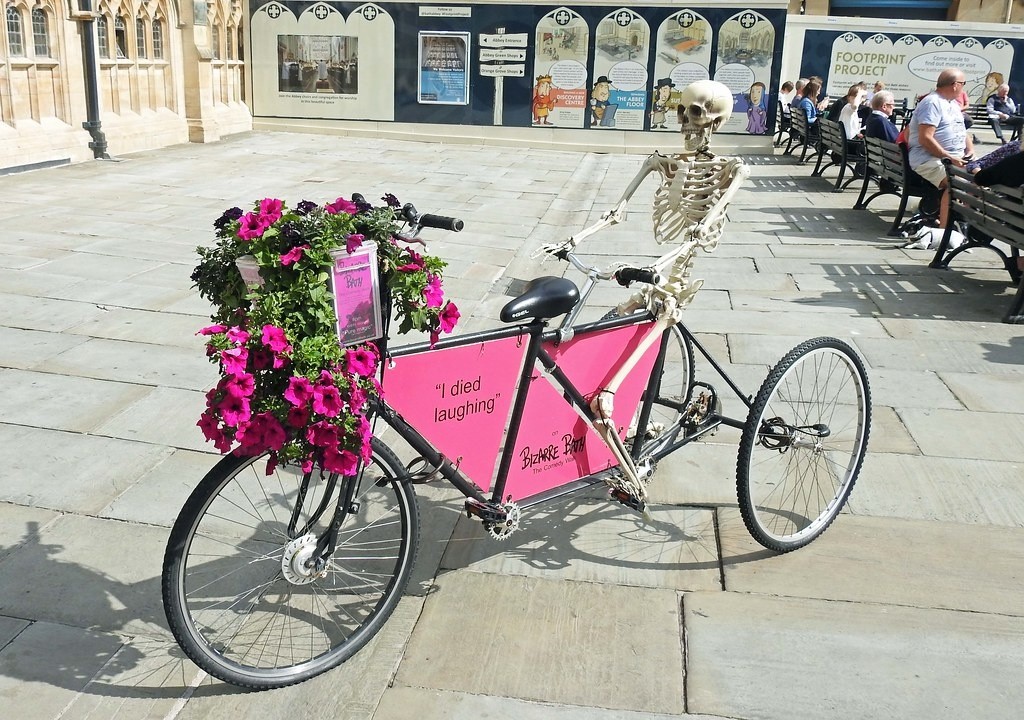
881 103 895 107
950 81 967 87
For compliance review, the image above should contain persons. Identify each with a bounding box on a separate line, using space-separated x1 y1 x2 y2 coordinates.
314 58 329 82
827 81 878 176
779 81 794 111
791 76 830 135
866 90 900 143
986 84 1024 145
332 59 358 69
908 68 976 229
955 90 974 129
895 93 929 150
282 58 310 81
869 81 896 125
966 126 1024 280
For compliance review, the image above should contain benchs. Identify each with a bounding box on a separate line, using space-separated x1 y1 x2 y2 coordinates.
774 93 1024 325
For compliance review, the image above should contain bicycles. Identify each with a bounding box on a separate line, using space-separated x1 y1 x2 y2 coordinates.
158 203 875 690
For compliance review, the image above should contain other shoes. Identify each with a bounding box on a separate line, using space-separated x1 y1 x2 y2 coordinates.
1002 139 1007 144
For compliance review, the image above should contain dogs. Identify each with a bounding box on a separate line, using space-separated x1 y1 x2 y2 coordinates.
893 221 973 255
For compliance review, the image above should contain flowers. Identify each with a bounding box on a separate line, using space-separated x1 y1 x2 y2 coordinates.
192 199 460 476
